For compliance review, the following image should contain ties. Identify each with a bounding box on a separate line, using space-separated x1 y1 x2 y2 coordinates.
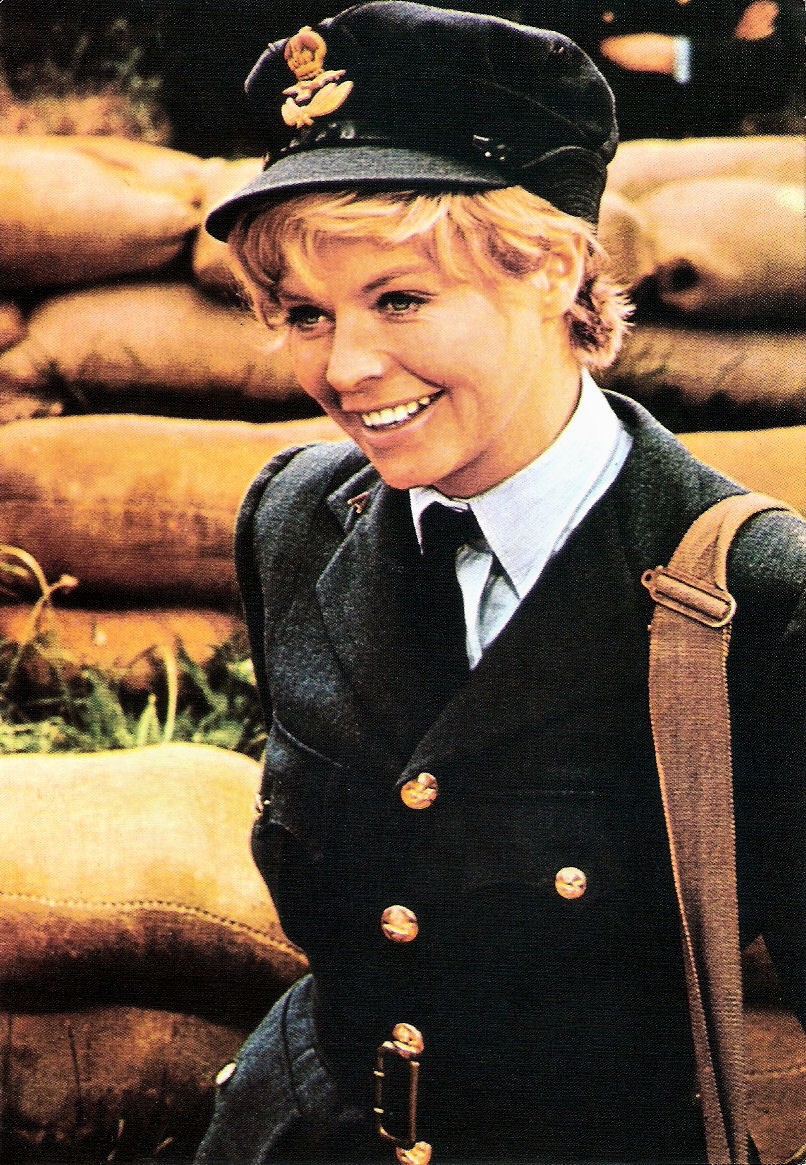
401 501 484 733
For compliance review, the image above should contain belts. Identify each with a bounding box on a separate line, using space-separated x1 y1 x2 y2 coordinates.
306 982 709 1165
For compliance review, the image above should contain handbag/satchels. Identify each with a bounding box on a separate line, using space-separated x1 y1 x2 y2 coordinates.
640 489 806 1165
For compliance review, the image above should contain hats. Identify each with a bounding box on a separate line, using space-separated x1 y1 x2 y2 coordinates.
204 1 620 242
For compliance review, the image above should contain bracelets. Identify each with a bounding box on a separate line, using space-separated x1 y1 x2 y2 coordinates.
672 34 691 84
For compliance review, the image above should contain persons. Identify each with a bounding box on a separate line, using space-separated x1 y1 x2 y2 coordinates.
519 0 806 141
193 0 806 1165
